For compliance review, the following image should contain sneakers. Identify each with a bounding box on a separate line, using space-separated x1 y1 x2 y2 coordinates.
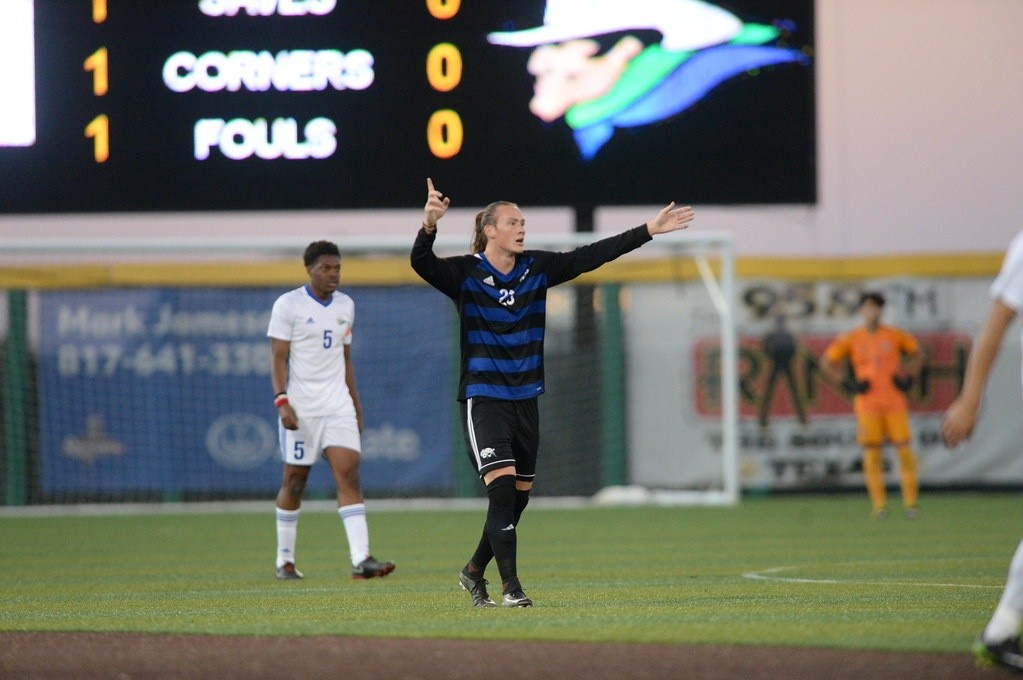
351 556 395 579
458 570 496 608
971 630 1023 676
501 590 533 609
274 562 303 579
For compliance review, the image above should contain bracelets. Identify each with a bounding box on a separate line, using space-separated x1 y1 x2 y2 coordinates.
272 391 288 408
423 221 436 228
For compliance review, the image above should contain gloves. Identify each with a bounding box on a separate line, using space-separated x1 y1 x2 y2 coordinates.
892 373 913 390
842 376 869 393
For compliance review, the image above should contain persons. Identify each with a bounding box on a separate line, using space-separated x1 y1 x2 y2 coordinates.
940 233 1023 667
814 292 923 519
268 241 396 579
760 313 806 430
410 177 695 609
